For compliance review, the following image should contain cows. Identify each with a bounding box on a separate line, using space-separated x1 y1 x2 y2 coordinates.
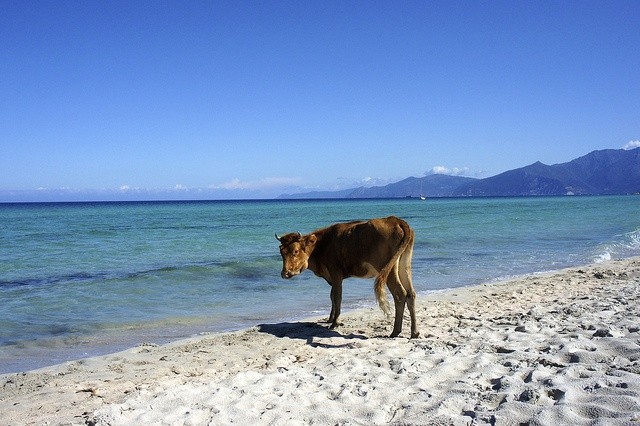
274 216 420 339
